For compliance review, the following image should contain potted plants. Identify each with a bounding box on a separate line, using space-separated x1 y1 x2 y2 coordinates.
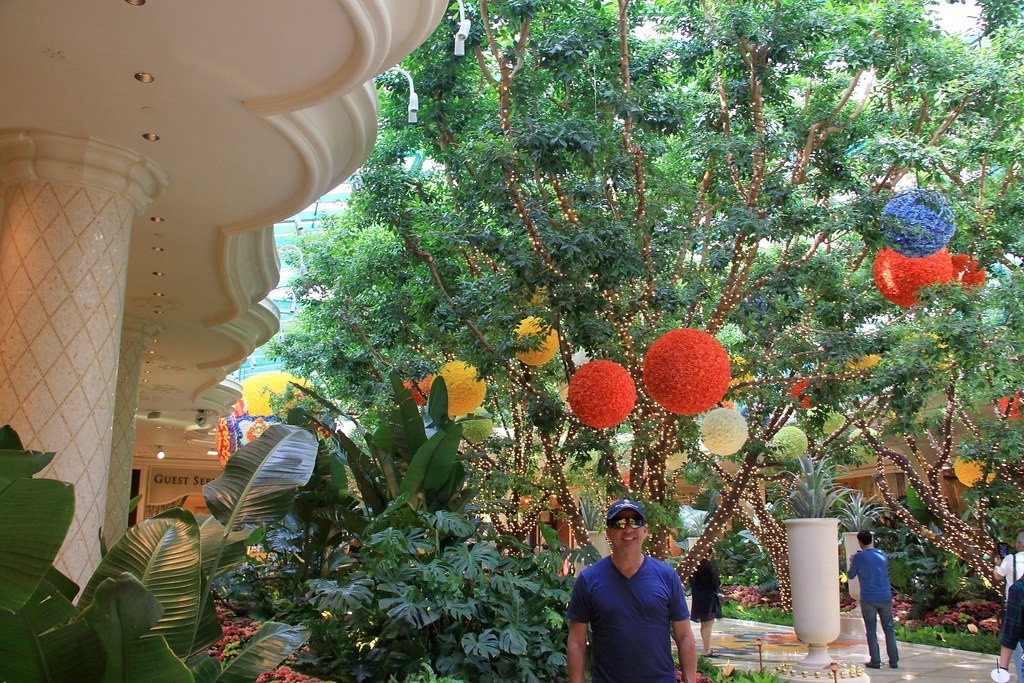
578 496 611 558
833 491 895 613
771 447 870 683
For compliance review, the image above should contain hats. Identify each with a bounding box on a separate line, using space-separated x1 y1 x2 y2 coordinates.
607 499 647 523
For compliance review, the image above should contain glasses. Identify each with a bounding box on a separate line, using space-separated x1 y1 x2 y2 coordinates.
606 516 646 529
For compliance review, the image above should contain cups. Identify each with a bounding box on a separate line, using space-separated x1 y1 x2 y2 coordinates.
991 668 1010 683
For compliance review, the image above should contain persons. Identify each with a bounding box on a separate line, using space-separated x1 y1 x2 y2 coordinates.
846 530 899 670
990 530 1024 678
564 500 698 683
689 547 723 657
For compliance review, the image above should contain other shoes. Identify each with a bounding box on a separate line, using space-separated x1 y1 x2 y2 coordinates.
889 661 898 668
865 662 880 668
702 649 720 657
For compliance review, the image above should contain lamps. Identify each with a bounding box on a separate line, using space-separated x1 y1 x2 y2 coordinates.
455 0 471 56
388 67 418 122
